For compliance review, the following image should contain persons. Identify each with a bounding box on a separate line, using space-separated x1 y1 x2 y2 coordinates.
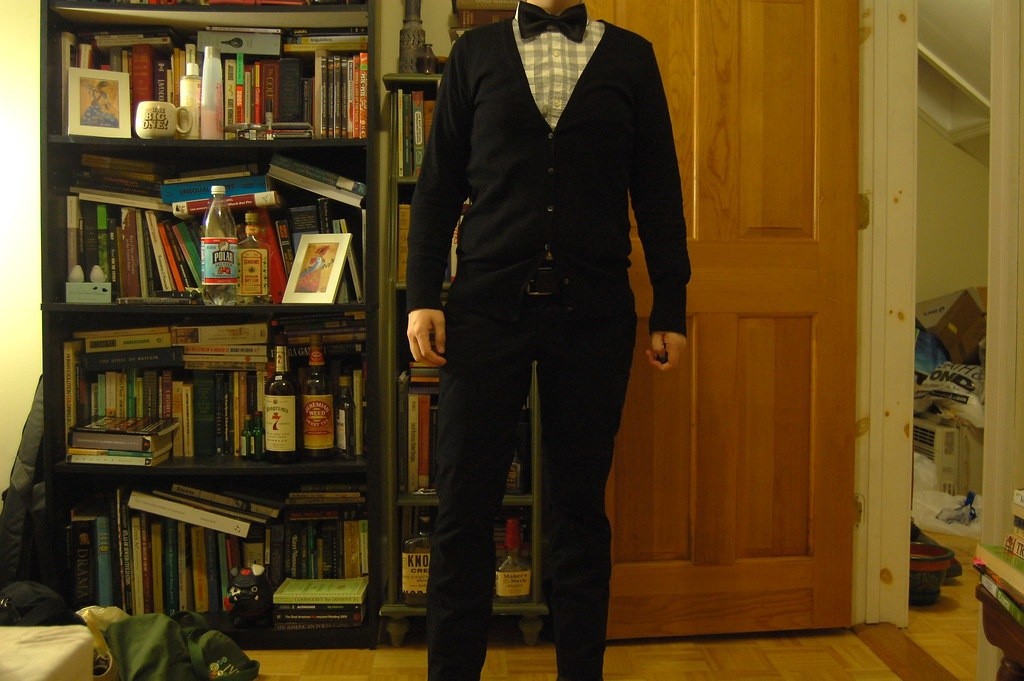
402 0 691 680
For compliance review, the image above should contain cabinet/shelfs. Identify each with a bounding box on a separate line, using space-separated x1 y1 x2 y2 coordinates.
38 0 550 646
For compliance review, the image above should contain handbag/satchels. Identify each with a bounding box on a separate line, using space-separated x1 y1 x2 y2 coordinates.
106 609 261 681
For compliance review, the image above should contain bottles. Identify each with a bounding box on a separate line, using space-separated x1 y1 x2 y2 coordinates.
241 414 252 459
402 516 431 608
179 62 200 140
301 333 336 461
200 186 239 306
496 517 533 604
250 410 265 461
504 436 525 494
335 375 357 461
262 334 303 463
236 212 272 305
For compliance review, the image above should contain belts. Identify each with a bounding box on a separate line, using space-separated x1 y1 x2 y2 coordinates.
526 266 574 295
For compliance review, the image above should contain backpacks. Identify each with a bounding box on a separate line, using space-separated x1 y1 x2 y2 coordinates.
0 580 87 627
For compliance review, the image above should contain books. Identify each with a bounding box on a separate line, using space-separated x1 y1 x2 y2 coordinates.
44 0 374 642
972 485 1024 626
393 86 530 496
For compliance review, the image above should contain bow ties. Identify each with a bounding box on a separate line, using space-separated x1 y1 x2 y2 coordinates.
517 1 587 44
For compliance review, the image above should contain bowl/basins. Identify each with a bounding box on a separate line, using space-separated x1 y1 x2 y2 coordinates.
910 542 956 607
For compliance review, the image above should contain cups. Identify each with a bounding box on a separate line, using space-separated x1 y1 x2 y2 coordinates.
134 101 194 140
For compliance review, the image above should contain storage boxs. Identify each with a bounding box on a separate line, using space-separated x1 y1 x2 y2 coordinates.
914 285 989 502
909 543 956 606
